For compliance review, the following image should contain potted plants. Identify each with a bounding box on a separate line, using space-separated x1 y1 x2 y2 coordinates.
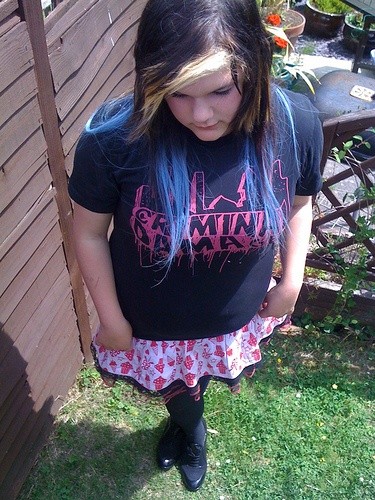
345 12 375 55
305 0 350 37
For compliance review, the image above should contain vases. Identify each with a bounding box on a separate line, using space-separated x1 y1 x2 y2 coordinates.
261 8 306 54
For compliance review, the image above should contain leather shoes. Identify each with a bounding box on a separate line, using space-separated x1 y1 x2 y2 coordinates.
156 420 180 470
181 416 207 490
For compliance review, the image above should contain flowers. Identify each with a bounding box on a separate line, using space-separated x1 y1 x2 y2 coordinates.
264 14 290 50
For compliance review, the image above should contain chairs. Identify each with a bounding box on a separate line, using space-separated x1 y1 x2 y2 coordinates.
313 69 375 170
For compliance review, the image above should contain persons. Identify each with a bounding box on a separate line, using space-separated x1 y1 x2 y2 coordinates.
67 0 325 492
